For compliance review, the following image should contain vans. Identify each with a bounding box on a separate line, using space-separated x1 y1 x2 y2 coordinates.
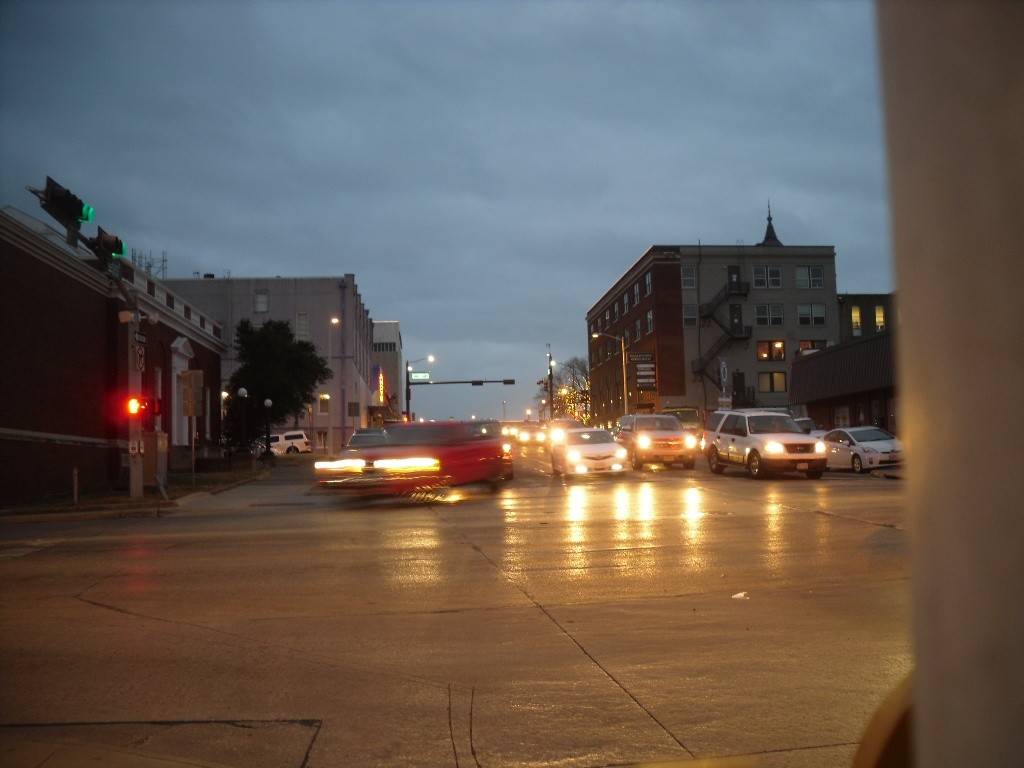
259 431 312 455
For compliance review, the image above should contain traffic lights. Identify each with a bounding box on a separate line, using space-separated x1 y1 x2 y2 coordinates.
96 224 127 258
43 175 94 228
127 397 143 414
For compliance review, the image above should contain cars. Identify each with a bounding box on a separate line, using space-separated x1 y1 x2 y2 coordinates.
314 419 586 502
550 427 628 478
819 426 903 473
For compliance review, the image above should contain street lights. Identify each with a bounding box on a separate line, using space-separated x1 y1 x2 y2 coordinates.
591 333 629 416
405 355 433 423
325 318 340 458
545 342 556 419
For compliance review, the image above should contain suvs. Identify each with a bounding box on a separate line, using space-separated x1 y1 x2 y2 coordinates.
614 416 696 469
661 408 703 440
701 411 827 478
793 418 829 440
